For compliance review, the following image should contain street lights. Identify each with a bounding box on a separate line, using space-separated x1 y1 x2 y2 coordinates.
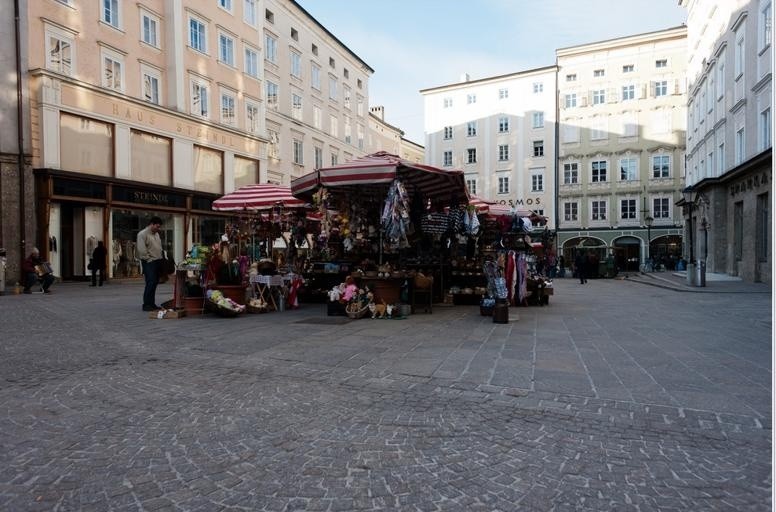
680 182 697 264
645 215 654 259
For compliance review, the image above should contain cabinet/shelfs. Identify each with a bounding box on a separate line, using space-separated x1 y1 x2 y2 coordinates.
408 249 448 305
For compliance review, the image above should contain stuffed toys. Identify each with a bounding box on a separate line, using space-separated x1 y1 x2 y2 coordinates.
211 289 241 311
339 276 358 304
327 285 344 302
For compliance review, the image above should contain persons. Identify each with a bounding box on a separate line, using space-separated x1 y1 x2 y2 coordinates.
113 238 122 273
89 241 108 287
88 233 97 259
137 216 164 313
126 239 135 261
652 253 686 270
49 233 57 265
22 249 54 294
575 249 587 284
558 256 564 277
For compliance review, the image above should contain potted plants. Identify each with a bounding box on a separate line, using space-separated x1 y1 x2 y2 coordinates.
361 258 379 278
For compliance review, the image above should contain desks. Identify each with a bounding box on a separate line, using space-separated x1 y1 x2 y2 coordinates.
252 275 283 312
355 277 405 319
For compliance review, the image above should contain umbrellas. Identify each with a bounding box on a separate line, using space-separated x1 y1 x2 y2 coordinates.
291 151 471 265
212 181 316 255
430 191 547 231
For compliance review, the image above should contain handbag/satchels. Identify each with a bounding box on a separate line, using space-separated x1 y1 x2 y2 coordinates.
150 258 168 276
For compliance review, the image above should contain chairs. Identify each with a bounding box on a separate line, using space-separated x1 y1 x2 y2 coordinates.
411 273 433 314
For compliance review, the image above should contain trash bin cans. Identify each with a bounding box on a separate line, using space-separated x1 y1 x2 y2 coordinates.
0 247 6 295
693 261 706 286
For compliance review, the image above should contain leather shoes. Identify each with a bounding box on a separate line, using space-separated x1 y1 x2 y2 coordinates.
143 305 152 311
151 304 161 309
